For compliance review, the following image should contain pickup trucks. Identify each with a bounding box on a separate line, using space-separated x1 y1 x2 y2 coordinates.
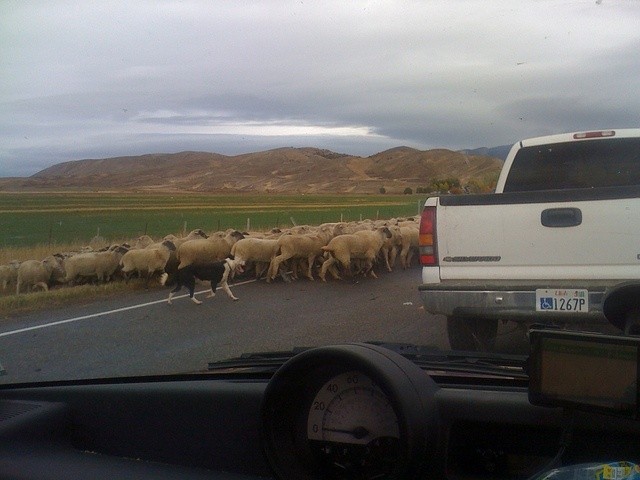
418 127 640 349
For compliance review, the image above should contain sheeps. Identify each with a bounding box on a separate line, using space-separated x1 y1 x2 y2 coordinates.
183 229 209 239
267 226 334 283
0 260 22 289
17 256 58 293
318 226 392 279
163 233 182 247
57 243 128 286
120 242 176 278
132 233 155 250
231 232 292 279
389 226 419 271
241 214 434 231
177 230 245 267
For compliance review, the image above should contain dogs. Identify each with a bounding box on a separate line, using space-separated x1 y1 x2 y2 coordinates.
161 256 247 304
